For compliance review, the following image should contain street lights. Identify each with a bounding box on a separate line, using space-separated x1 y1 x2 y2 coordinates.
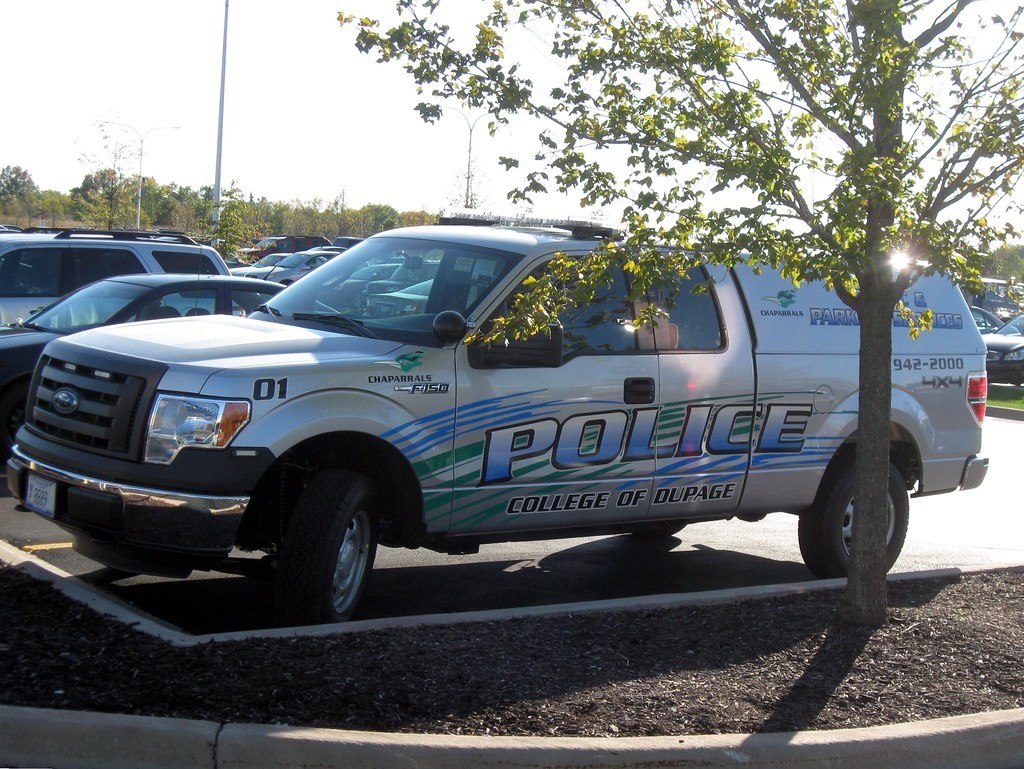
102 121 181 229
442 106 499 209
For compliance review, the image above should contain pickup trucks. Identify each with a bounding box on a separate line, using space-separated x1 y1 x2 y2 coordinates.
5 218 988 628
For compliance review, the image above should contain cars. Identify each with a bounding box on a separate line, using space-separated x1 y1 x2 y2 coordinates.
969 306 1005 332
224 235 442 320
0 274 340 464
954 278 1024 322
982 313 1024 386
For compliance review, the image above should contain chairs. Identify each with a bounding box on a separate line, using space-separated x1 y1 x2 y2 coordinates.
185 308 210 317
150 306 181 321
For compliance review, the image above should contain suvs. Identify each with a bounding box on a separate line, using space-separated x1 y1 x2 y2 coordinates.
0 225 230 326
